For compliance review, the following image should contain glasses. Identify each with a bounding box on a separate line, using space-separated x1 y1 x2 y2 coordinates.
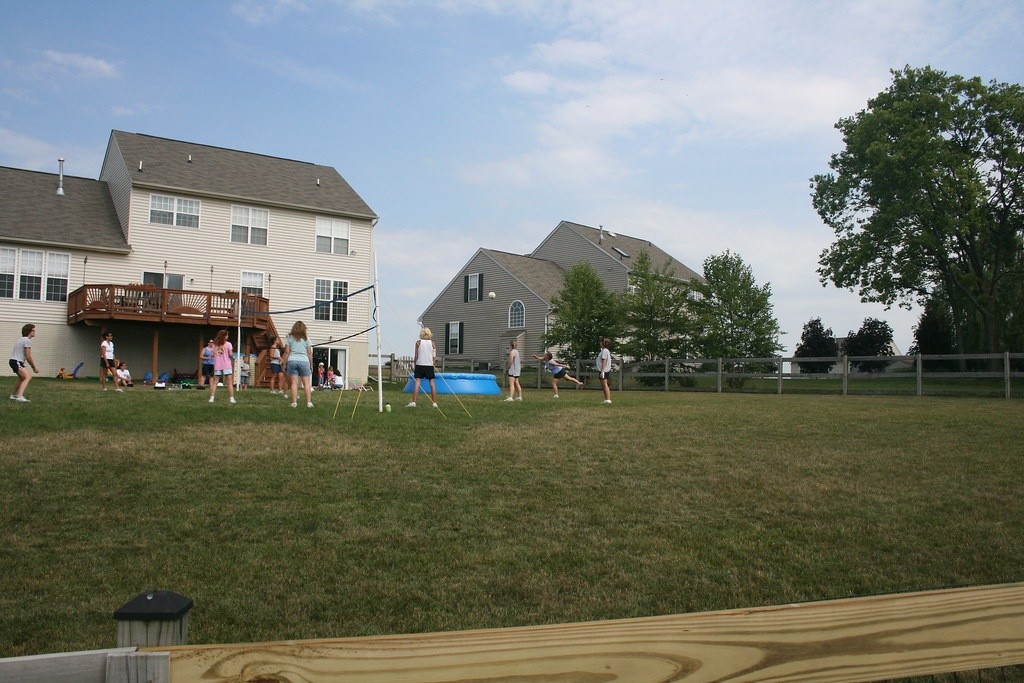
209 342 214 343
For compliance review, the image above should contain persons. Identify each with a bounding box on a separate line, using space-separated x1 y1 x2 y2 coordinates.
282 321 314 408
100 332 122 392
208 330 237 403
116 361 132 386
269 336 284 393
327 366 333 385
318 362 324 384
8 324 39 402
505 340 523 402
406 327 438 408
241 356 250 391
533 352 583 398
596 338 612 403
200 339 214 388
330 370 343 390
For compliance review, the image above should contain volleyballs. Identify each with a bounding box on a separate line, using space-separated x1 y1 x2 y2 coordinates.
488 292 496 299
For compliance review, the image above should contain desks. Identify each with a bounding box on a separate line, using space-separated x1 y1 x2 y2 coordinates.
113 295 162 313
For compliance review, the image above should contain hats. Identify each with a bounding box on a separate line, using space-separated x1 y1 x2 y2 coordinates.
319 362 324 366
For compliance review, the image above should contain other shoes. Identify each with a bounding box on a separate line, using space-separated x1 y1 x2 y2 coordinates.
297 396 300 399
576 382 582 385
209 396 214 402
270 391 276 393
601 400 611 404
514 397 522 401
405 402 416 407
290 403 298 407
432 402 438 407
10 394 16 399
504 397 514 402
284 394 287 398
102 388 107 391
15 396 30 402
229 397 236 403
553 394 560 399
115 388 123 392
306 402 314 407
279 391 283 393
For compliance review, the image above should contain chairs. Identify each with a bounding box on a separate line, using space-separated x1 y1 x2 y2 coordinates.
122 283 161 313
